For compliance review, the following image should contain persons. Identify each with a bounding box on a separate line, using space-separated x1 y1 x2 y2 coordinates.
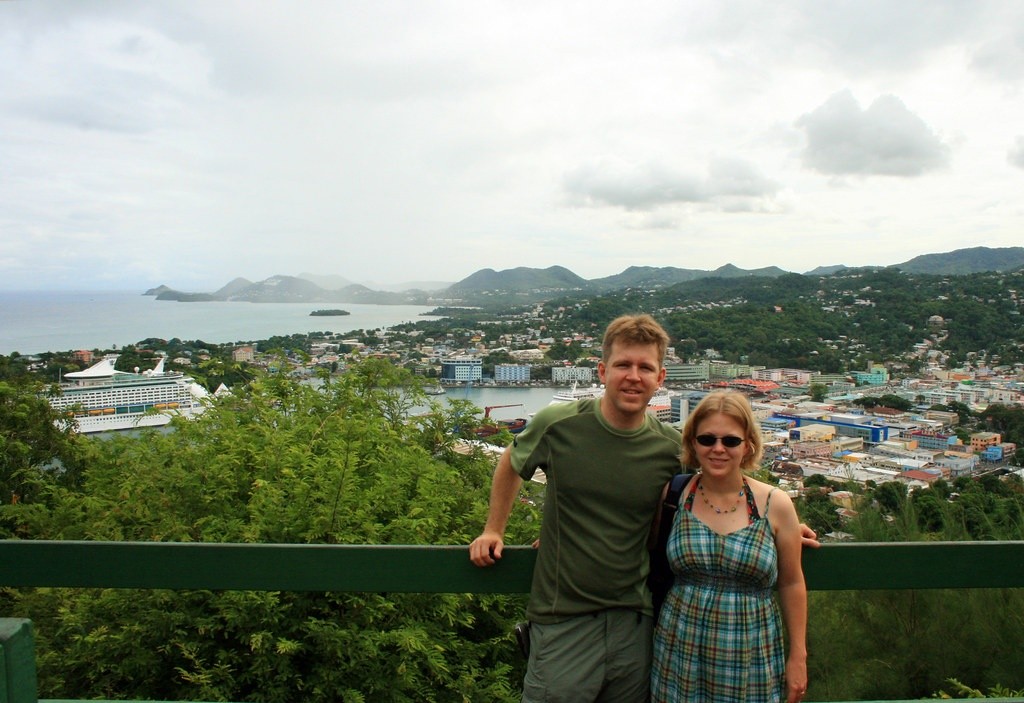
532 391 807 703
468 314 820 703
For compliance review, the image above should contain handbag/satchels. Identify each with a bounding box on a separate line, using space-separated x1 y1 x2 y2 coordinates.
649 473 693 626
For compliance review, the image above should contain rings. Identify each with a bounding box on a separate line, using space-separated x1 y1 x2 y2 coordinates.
801 692 804 694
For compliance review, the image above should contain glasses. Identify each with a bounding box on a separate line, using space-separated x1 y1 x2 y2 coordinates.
695 434 746 448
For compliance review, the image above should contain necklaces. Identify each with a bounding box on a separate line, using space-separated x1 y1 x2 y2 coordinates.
698 482 744 514
